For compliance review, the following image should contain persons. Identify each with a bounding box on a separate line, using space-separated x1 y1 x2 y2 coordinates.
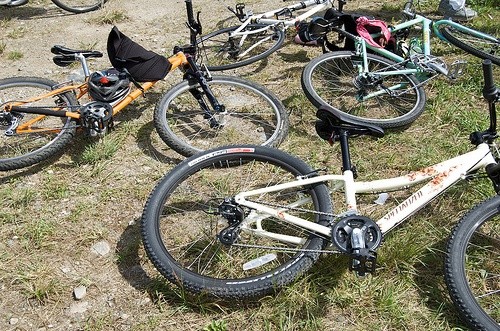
436 0 479 23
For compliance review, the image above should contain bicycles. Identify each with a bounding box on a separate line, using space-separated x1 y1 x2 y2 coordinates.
0 0 500 172
139 57 500 331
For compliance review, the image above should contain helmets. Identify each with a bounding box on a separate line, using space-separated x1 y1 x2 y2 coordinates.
89 69 130 102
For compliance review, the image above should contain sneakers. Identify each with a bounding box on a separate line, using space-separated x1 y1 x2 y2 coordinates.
437 6 449 15
444 6 477 21
